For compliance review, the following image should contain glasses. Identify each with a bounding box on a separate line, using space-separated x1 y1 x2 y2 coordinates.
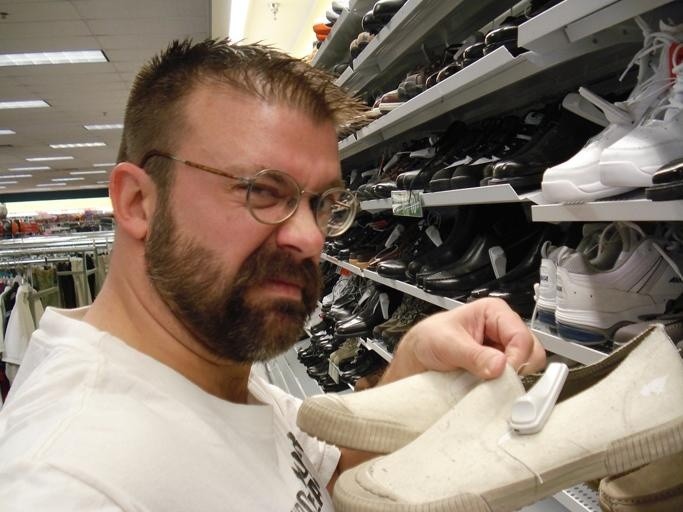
138 150 360 240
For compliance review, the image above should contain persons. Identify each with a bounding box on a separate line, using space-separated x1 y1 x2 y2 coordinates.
0 33 550 511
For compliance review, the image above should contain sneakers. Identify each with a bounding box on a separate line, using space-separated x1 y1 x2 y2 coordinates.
299 1 681 511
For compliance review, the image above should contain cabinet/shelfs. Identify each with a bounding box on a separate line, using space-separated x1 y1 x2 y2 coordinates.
267 0 683 512
